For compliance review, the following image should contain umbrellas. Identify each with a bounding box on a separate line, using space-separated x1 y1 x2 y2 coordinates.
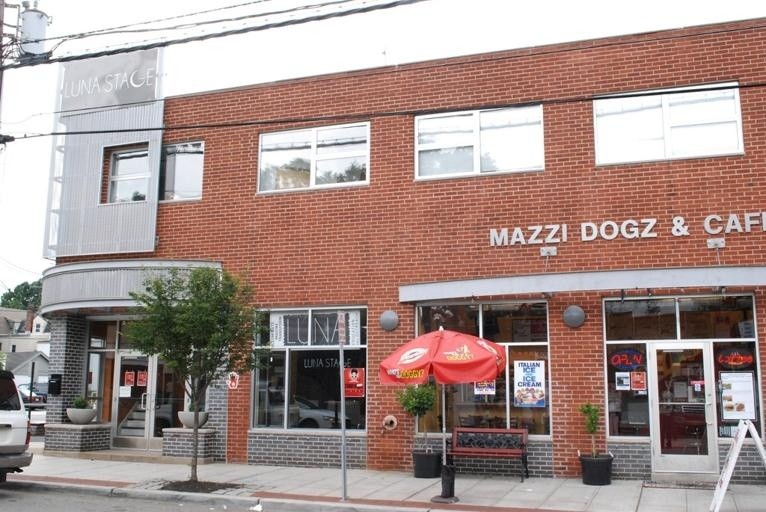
378 326 506 466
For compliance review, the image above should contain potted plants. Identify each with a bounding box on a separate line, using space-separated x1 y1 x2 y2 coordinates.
178 403 209 428
395 384 442 478
578 399 615 486
66 396 99 425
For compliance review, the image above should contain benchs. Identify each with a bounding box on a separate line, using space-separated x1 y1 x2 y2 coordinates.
446 427 528 483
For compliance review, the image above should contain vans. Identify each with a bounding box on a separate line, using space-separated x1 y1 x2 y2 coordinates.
259 389 299 429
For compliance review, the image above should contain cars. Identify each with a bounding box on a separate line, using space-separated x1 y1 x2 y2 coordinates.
0 369 33 482
155 404 173 436
19 389 43 410
292 388 352 429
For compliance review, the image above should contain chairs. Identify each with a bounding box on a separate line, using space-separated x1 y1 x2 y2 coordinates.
458 415 549 433
